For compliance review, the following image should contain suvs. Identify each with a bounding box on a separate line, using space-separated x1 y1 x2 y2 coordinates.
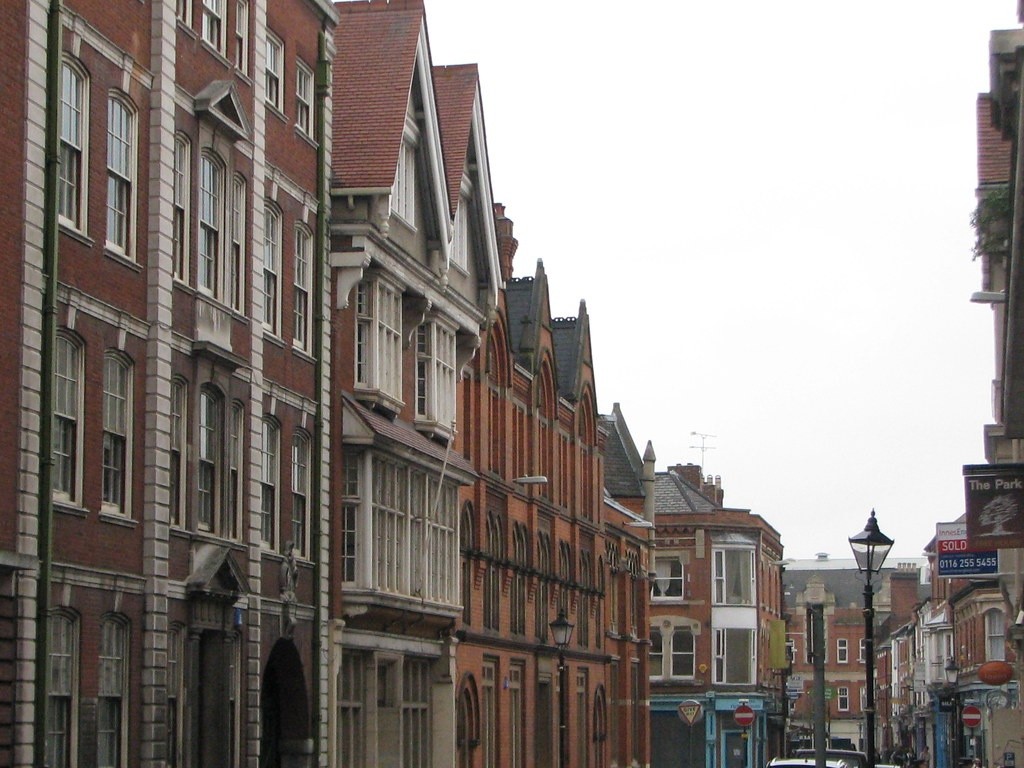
766 757 848 768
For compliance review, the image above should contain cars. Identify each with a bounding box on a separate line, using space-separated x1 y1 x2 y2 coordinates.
792 749 867 768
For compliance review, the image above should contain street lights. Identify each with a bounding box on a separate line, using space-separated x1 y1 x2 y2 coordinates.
549 608 574 768
848 507 896 768
945 661 959 768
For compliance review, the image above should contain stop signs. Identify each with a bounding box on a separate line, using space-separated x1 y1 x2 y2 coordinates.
734 705 755 726
962 706 981 727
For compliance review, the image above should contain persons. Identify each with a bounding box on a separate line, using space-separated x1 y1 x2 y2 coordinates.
956 757 981 768
873 742 930 768
